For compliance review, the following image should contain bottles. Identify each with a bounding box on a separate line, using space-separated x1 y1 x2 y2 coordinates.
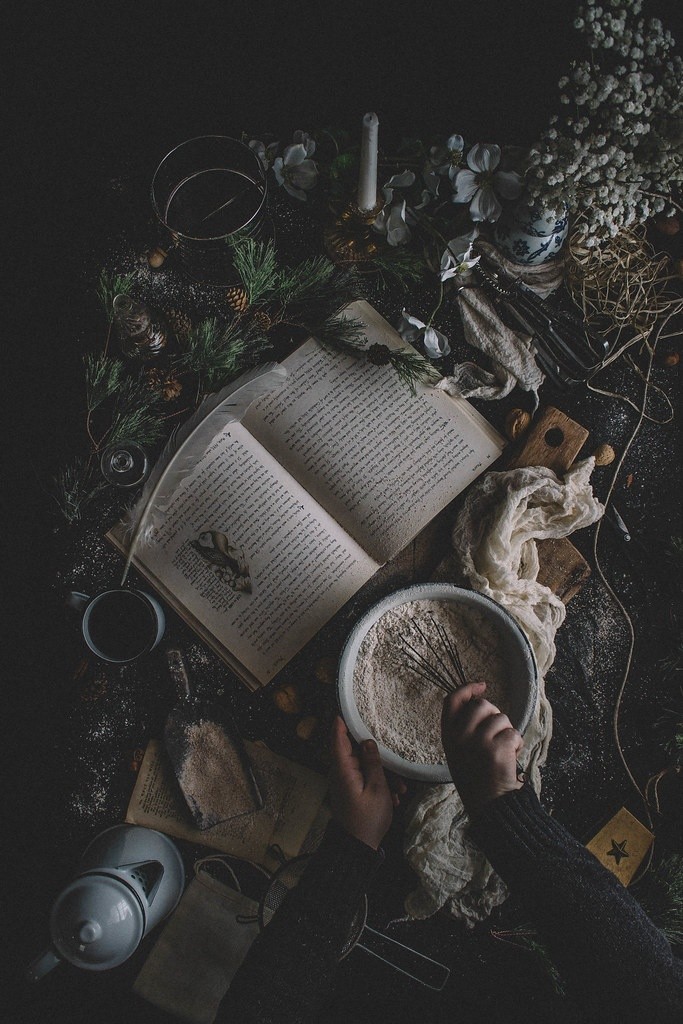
113 294 166 355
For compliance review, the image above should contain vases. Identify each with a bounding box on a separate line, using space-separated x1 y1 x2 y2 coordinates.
496 194 569 265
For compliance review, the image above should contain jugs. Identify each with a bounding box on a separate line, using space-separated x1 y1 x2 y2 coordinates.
28 826 185 983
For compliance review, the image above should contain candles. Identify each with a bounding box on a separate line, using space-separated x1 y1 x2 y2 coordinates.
357 110 379 212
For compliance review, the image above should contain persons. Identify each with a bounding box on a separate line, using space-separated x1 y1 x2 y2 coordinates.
215 681 683 1024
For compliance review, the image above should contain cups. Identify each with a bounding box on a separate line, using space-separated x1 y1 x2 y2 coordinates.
68 585 165 664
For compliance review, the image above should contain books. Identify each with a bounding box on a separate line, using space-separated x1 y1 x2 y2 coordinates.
122 736 330 877
102 297 508 692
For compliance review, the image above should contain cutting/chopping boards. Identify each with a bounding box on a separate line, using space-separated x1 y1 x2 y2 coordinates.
303 406 592 687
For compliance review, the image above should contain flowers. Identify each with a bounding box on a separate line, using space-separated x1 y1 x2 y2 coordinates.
525 0 683 248
245 125 520 360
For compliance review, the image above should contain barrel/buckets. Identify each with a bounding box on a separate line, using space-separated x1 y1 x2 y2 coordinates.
150 135 276 288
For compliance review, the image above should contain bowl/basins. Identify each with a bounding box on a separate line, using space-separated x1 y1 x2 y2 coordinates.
336 582 538 783
